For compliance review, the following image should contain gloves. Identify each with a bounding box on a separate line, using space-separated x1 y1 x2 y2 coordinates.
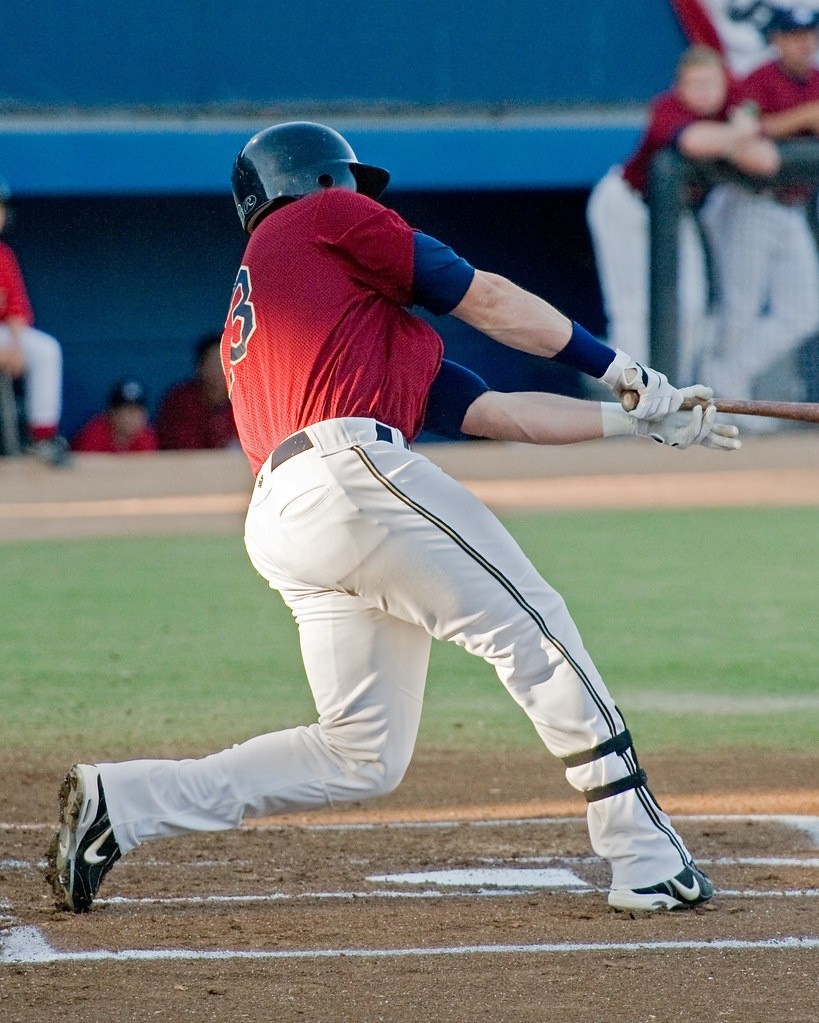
600 347 683 423
599 385 743 450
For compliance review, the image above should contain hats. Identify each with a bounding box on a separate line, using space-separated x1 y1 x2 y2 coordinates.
776 8 819 30
109 380 145 408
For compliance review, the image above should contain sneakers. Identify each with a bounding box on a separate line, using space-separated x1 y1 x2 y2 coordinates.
608 861 711 911
48 762 120 913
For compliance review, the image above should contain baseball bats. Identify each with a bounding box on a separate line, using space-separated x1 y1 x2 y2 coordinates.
616 390 819 426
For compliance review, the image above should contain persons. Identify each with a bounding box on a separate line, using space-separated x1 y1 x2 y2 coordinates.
154 334 242 451
586 40 780 403
75 377 160 453
699 2 819 429
0 175 73 468
45 119 741 912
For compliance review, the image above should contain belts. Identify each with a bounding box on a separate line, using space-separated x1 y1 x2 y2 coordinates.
272 424 409 474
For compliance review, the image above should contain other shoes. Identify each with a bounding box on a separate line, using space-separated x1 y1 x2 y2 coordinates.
25 439 64 464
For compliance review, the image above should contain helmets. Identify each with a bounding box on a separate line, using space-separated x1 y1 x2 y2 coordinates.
229 122 392 236
